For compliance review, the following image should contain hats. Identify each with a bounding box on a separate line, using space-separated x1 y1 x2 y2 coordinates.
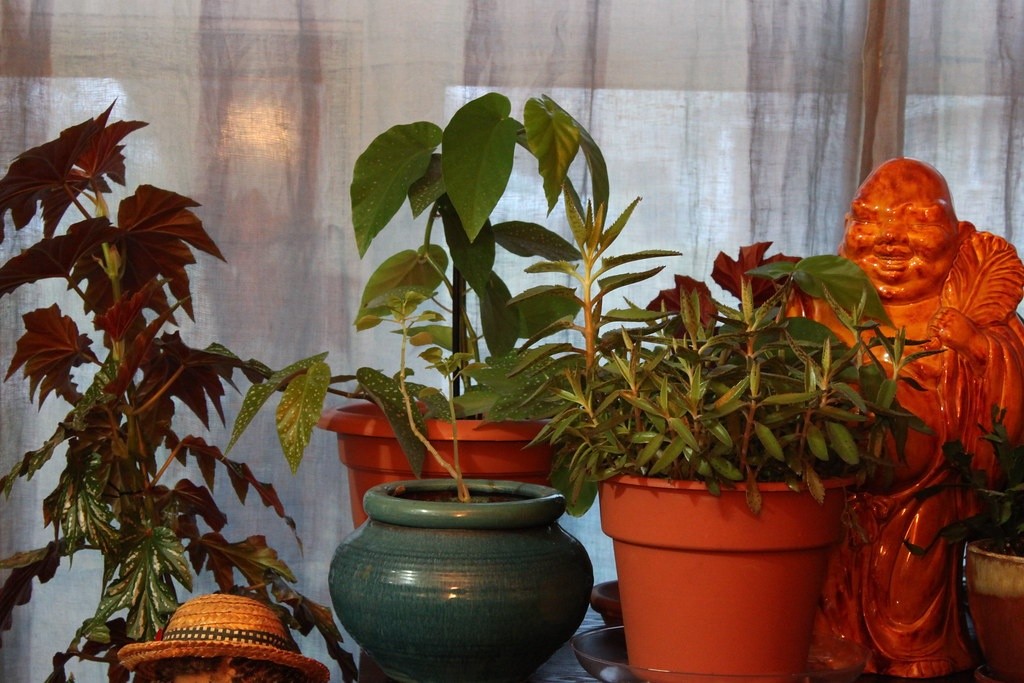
117 594 332 683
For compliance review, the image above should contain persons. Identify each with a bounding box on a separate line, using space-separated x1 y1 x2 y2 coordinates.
788 157 1024 678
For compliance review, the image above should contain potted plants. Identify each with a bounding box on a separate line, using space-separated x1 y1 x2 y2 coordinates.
1 90 1024 683
